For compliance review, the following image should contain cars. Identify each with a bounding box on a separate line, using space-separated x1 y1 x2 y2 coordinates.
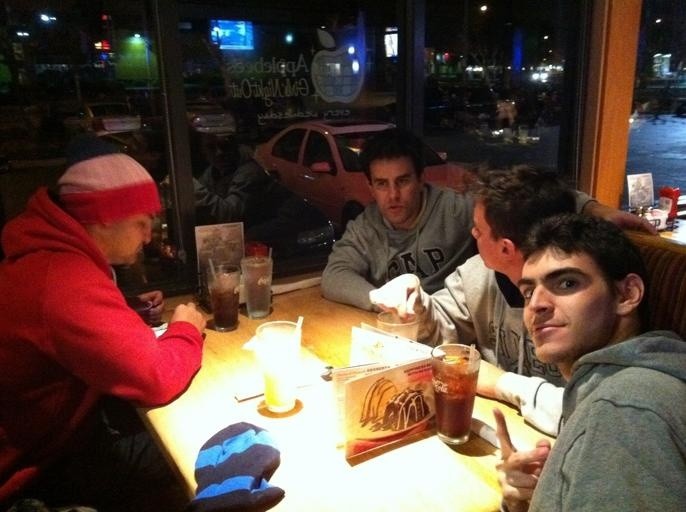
251 117 487 241
421 74 573 132
184 98 238 142
54 127 337 294
58 98 141 140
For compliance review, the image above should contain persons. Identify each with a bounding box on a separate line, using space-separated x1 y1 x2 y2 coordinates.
368 160 577 440
317 126 658 314
490 213 685 512
0 152 209 512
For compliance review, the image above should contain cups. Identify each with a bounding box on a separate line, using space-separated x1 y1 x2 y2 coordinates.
255 316 300 413
205 262 241 331
125 295 152 329
430 341 483 447
377 306 422 344
238 255 273 320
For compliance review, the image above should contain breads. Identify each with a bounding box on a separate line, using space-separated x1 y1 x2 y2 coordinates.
383 388 428 430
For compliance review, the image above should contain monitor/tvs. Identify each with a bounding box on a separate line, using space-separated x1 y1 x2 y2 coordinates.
383 32 399 58
208 19 255 52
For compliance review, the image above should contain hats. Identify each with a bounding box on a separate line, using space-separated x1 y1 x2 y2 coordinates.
188 420 287 512
50 151 167 226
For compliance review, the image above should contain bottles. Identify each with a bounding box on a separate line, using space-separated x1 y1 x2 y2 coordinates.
643 205 654 228
658 186 682 221
635 205 644 220
243 242 270 261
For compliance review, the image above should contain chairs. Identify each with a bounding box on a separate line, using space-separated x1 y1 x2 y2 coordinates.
135 282 557 511
620 226 686 344
